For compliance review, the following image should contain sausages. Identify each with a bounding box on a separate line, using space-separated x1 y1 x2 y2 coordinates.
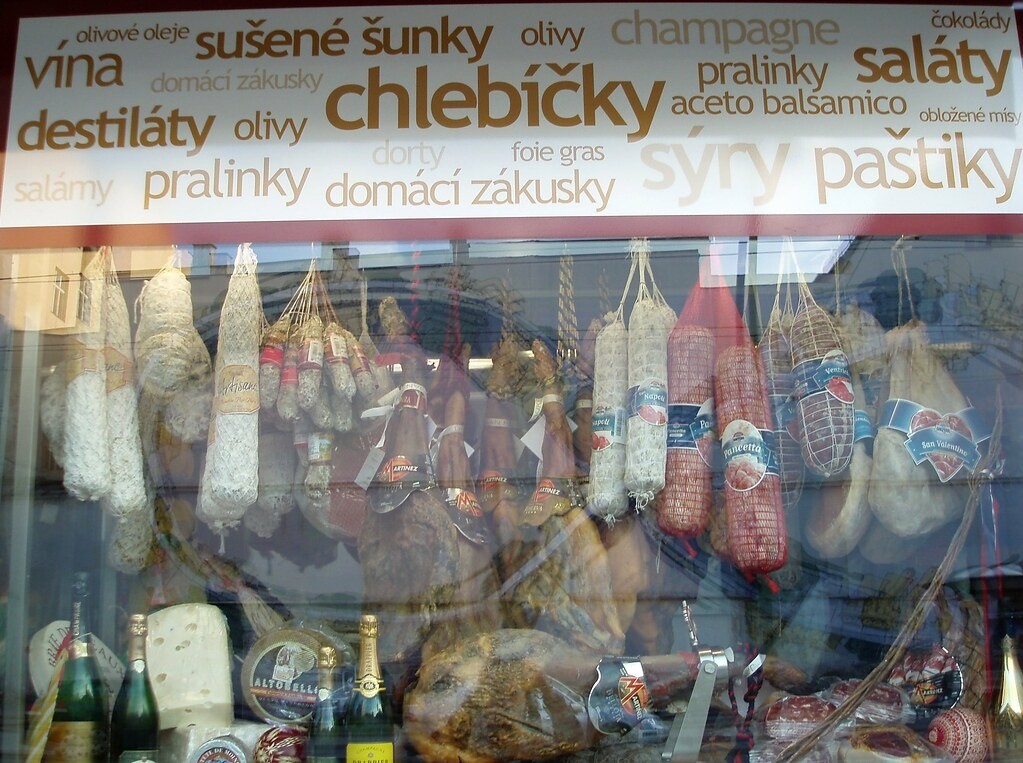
42 249 851 575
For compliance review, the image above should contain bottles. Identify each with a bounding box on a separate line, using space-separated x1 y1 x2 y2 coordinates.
306 647 344 763
46 572 108 763
345 615 394 763
110 613 160 763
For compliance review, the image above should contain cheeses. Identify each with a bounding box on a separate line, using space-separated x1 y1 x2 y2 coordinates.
146 603 275 763
